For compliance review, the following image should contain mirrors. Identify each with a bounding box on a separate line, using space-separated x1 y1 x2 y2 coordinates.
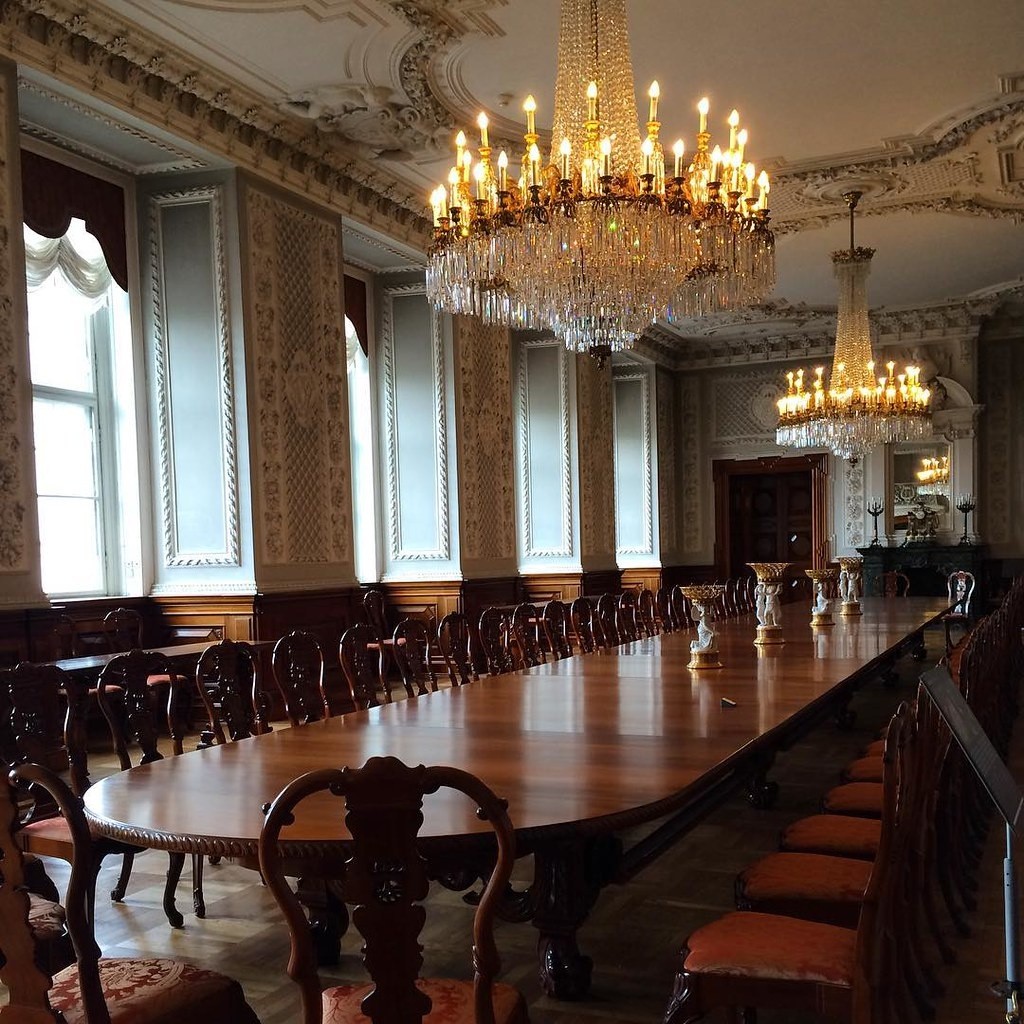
884 432 956 535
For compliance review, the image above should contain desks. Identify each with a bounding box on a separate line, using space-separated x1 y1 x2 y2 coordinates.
30 633 280 676
104 573 960 1007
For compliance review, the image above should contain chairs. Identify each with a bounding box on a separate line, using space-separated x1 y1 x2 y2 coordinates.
568 596 599 656
780 574 1024 962
196 636 274 750
1 758 264 1024
542 598 574 660
256 754 526 1024
437 610 479 688
272 629 332 729
735 670 949 1017
42 612 131 753
594 573 760 644
0 658 188 929
104 605 193 734
361 589 419 679
338 620 392 713
654 700 940 1024
511 602 548 668
476 605 516 675
97 647 224 917
391 619 438 699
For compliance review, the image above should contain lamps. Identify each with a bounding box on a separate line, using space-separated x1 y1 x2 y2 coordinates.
916 452 950 498
422 0 787 378
866 495 886 549
956 490 977 547
771 188 937 474
